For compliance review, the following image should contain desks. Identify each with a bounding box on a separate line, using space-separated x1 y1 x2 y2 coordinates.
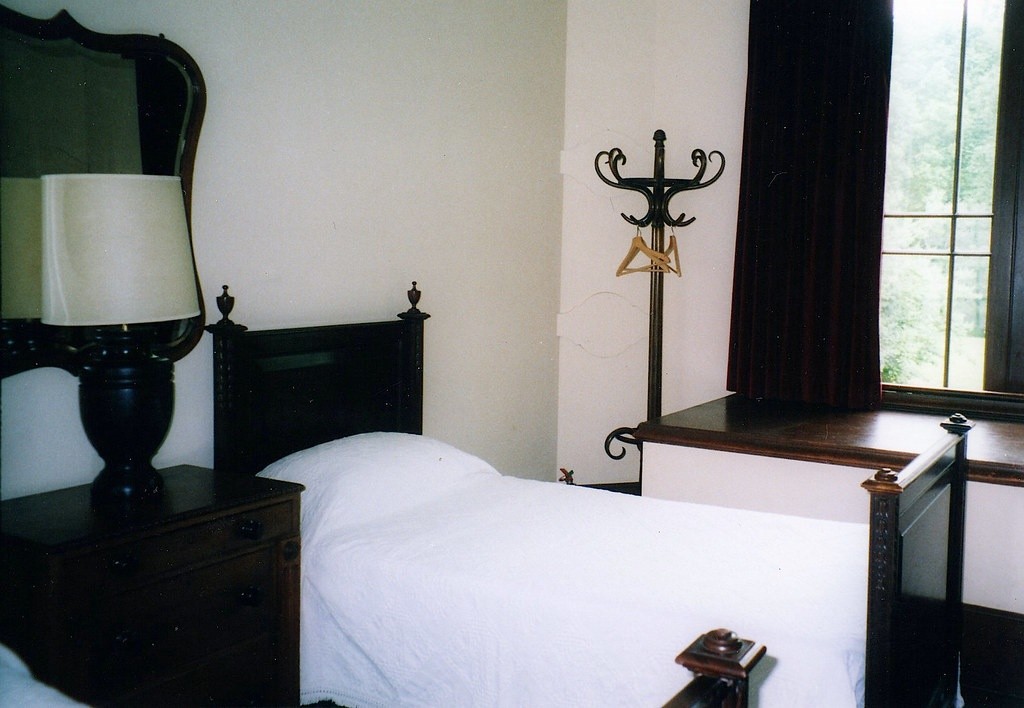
634 392 1024 641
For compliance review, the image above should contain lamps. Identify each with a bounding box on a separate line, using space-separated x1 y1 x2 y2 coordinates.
41 174 200 516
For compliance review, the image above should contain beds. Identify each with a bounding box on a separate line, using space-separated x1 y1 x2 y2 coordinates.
205 282 976 708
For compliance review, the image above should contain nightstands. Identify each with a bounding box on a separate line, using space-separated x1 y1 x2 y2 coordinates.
1 464 305 707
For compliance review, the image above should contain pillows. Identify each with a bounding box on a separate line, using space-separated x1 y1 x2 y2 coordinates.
255 432 496 551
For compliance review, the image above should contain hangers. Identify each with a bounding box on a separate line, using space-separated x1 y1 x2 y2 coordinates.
616 224 682 277
